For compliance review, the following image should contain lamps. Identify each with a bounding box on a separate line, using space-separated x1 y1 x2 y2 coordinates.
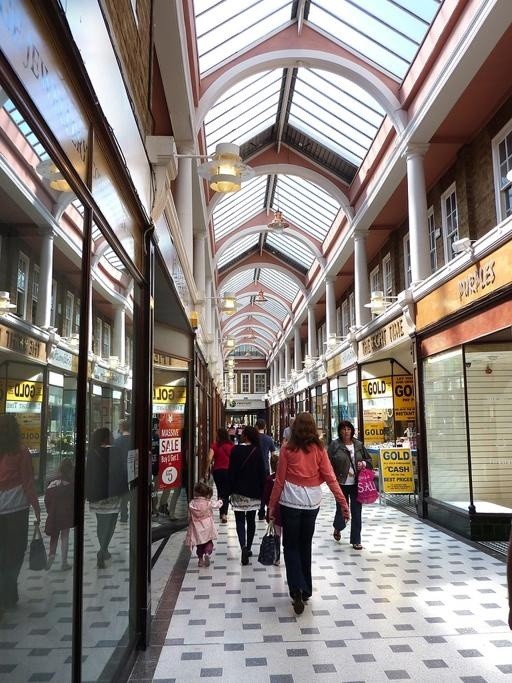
267 172 290 236
143 132 257 225
201 289 238 383
321 290 399 366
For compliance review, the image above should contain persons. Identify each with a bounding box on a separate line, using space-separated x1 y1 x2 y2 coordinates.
205 427 235 525
255 418 276 520
282 416 296 444
261 454 283 567
265 411 350 615
0 412 182 614
226 424 246 445
222 426 268 568
182 482 224 567
325 420 373 550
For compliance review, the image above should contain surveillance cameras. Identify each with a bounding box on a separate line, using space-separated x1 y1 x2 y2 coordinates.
452 237 478 257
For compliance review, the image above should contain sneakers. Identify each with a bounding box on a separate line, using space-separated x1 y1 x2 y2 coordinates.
95 552 117 566
47 558 71 571
242 546 252 564
199 555 210 566
292 588 311 614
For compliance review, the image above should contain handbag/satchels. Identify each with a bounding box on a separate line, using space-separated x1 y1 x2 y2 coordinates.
357 468 378 504
258 534 280 565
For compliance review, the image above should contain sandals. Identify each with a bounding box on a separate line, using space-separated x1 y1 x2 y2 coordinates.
352 543 362 549
334 529 341 541
